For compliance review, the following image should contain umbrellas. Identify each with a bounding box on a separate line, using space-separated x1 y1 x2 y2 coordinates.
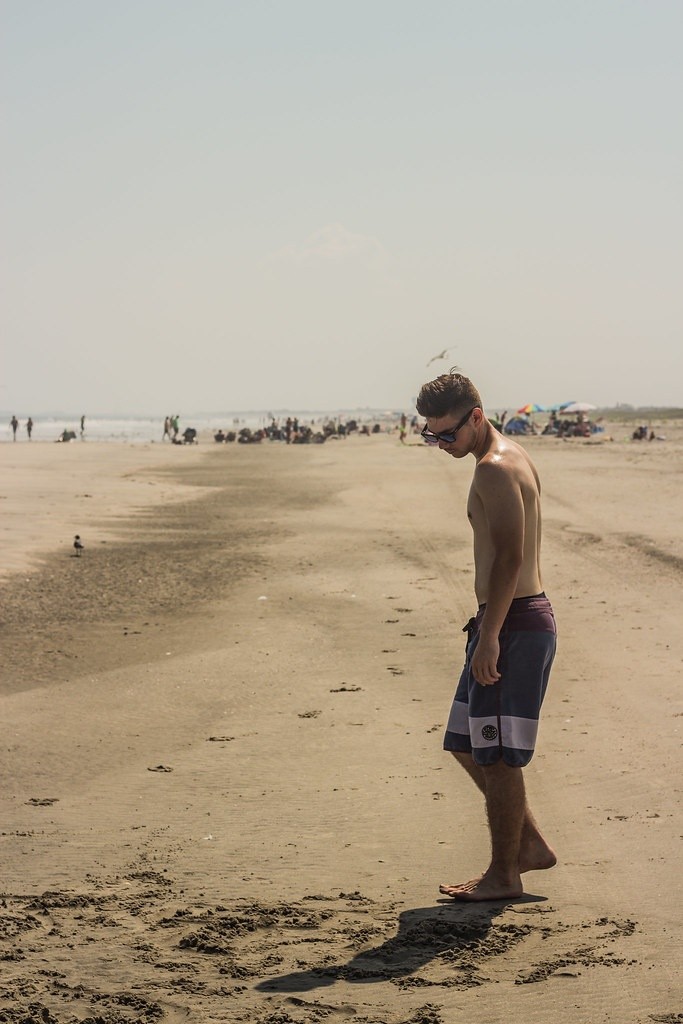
518 401 598 415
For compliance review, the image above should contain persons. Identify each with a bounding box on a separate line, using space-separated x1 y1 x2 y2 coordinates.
161 410 667 445
416 367 558 903
27 418 33 442
10 416 18 443
80 416 85 436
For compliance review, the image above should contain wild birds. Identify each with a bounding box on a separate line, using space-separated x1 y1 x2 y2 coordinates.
72 535 84 557
426 349 448 368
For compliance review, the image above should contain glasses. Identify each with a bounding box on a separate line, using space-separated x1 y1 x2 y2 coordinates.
420 405 481 443
